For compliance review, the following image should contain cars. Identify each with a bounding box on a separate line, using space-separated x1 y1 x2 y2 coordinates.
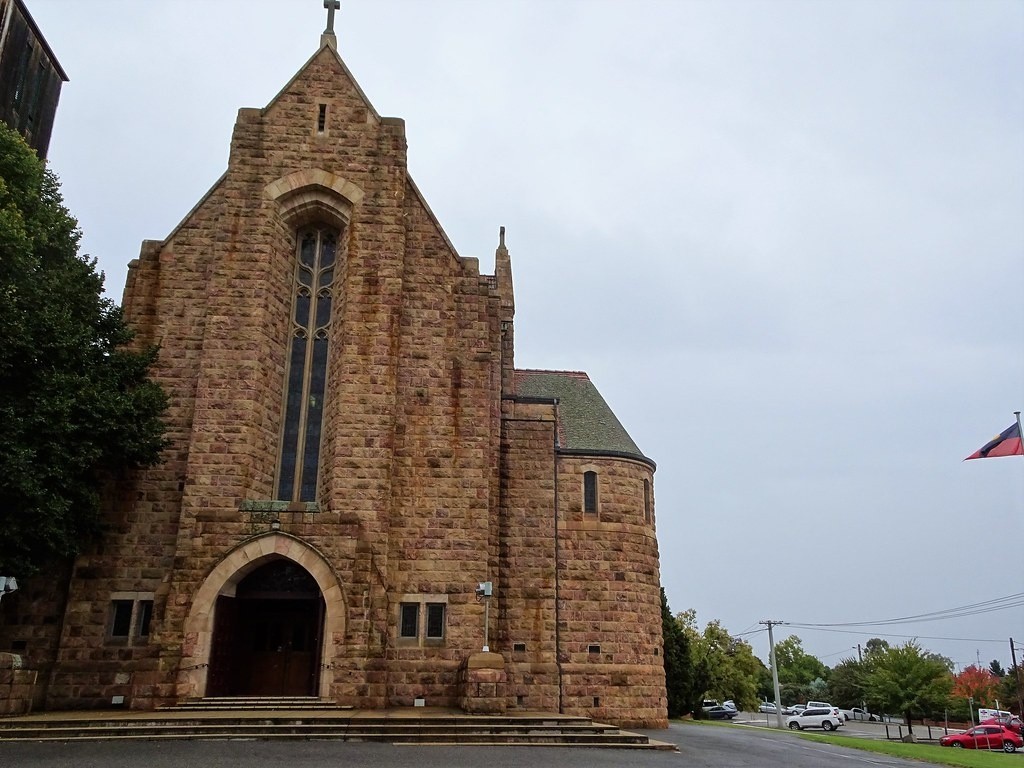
787 703 805 715
981 716 1024 725
722 700 736 708
707 706 738 720
939 716 1024 753
758 702 785 715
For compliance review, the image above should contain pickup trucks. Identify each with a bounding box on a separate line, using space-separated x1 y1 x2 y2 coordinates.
840 708 880 722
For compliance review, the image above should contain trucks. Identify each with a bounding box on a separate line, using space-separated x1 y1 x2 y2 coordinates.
979 708 1011 724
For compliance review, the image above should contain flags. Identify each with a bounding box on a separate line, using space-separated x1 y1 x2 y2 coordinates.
964 423 1024 460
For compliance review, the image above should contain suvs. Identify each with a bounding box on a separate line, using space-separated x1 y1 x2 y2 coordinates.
702 700 721 712
785 707 846 731
806 701 832 713
